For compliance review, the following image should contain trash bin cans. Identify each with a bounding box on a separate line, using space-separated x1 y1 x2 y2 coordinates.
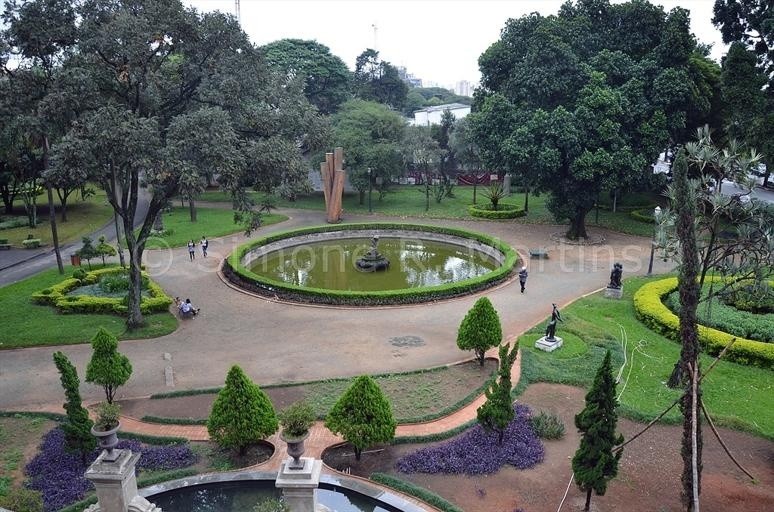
71 255 79 266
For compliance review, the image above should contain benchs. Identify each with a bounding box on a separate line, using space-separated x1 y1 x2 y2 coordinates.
174 298 195 320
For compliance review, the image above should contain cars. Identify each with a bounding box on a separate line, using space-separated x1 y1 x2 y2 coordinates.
749 164 766 176
767 177 774 188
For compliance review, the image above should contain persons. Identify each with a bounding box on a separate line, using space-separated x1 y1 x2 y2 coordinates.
184 297 199 314
544 304 564 339
607 266 621 287
174 297 183 306
199 235 208 258
609 261 623 286
517 266 528 294
186 239 195 263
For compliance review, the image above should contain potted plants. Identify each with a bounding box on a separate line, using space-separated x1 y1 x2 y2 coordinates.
90 401 123 462
278 400 317 470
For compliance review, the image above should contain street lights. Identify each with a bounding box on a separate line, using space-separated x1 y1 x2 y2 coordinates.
366 167 371 214
646 205 662 275
